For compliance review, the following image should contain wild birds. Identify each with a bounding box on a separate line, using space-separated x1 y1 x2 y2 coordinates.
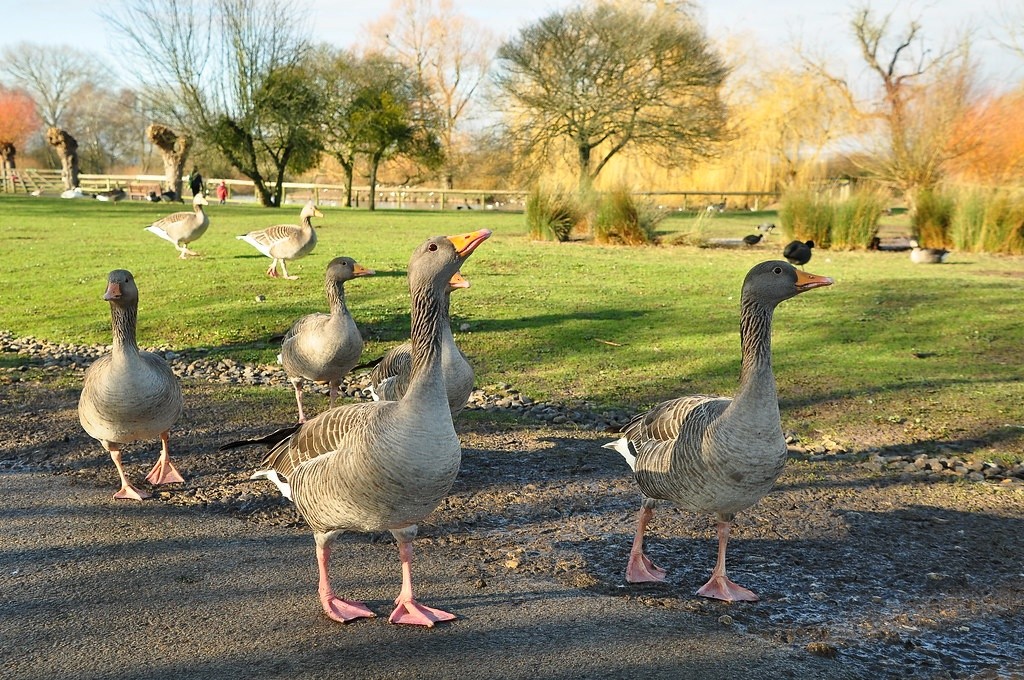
601 260 832 606
743 223 775 248
78 269 186 502
909 240 950 264
235 204 323 281
784 240 815 272
219 228 492 627
142 192 209 260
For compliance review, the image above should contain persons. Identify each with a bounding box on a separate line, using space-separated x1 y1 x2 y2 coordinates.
217 181 229 205
186 165 204 198
